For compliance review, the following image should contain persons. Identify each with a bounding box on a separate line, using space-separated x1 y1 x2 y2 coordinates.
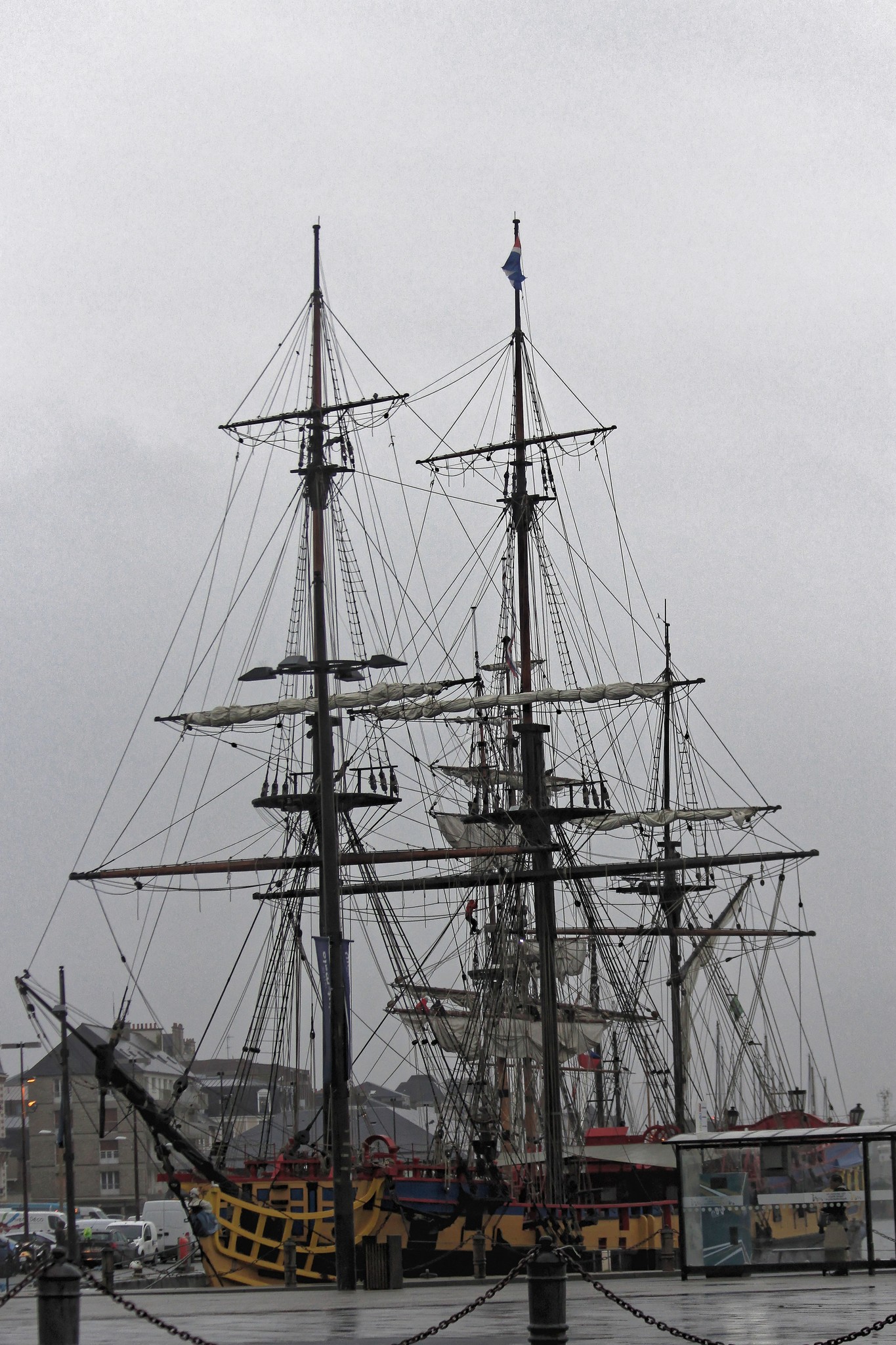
821 1175 852 1277
55 1221 65 1247
465 900 479 935
14 1240 23 1257
414 996 448 1031
188 1189 212 1214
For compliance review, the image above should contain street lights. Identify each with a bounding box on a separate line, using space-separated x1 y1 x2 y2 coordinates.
53 965 80 1271
725 1086 865 1130
1 1041 42 1243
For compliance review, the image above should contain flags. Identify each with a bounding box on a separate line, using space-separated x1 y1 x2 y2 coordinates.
500 234 528 300
577 1050 603 1071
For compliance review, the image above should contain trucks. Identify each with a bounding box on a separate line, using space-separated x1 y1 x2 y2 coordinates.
0 1200 201 1278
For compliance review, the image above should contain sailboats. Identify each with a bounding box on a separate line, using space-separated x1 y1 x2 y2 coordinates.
13 212 816 1287
